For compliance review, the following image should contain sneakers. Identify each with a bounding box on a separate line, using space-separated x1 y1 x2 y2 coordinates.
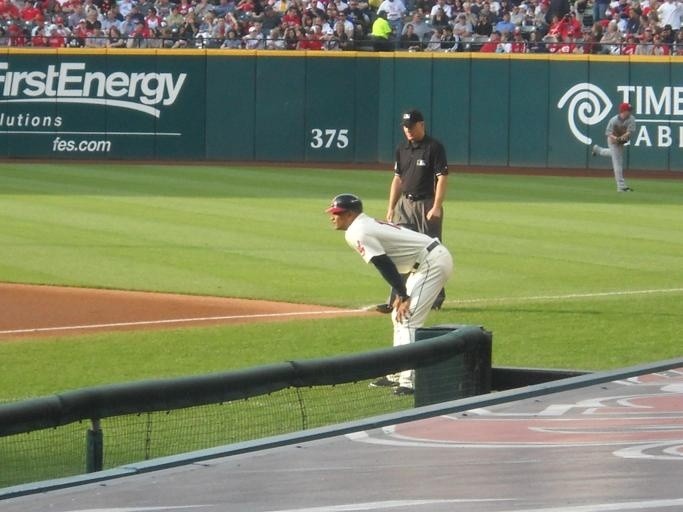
376 303 393 314
615 185 634 193
370 378 414 395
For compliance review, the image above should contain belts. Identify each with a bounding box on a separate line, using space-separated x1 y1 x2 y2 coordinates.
402 191 432 201
413 241 438 270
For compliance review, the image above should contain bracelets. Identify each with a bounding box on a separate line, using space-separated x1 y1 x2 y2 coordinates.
398 296 409 303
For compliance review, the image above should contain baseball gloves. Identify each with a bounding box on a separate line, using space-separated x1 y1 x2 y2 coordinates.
616 136 630 144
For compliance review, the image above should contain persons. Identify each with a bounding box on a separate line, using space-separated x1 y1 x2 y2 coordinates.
590 103 637 192
373 109 449 316
322 192 454 398
2 1 683 56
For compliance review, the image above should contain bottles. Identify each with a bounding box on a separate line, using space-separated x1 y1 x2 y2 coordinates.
672 40 678 56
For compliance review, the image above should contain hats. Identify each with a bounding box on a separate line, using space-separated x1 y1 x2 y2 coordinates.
619 103 634 112
399 110 423 128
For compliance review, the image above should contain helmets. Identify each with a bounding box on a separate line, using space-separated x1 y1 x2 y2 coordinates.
325 194 363 216
